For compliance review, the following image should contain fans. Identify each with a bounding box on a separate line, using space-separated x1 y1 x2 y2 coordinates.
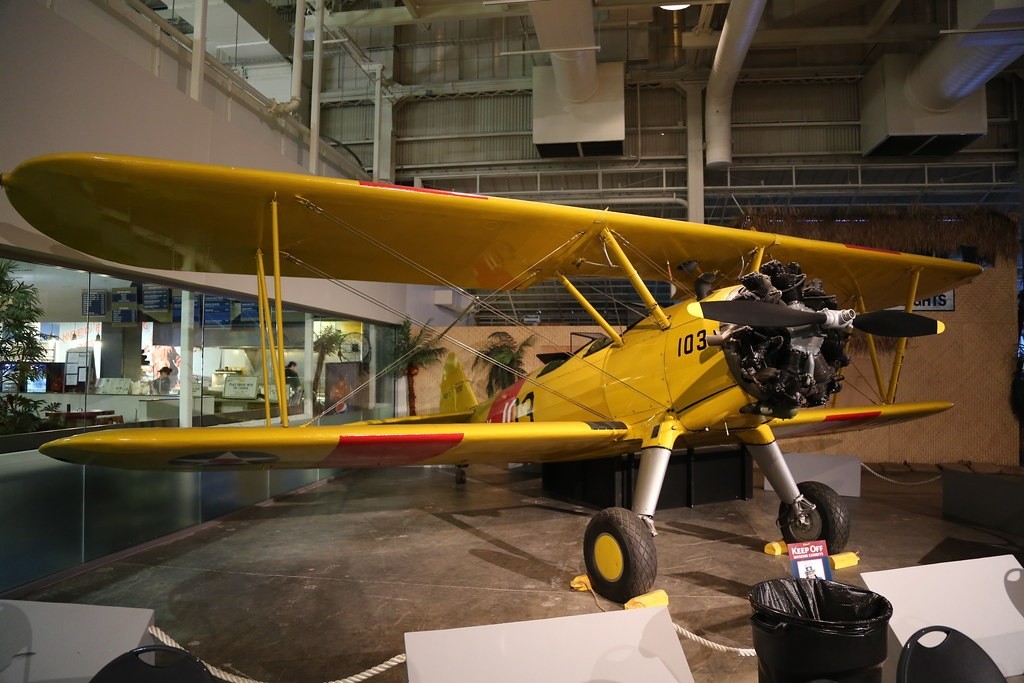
337 332 370 362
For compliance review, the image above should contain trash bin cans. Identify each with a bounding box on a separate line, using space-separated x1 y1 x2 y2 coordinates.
747 578 893 683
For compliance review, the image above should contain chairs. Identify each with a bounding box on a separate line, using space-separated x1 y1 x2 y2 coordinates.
87 644 218 682
893 626 1005 683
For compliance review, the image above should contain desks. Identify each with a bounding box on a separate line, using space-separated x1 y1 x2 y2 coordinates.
46 409 116 427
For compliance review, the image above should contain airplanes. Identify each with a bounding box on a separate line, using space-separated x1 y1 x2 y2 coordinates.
0 153 985 604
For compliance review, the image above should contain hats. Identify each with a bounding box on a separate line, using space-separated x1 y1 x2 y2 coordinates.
157 367 170 373
284 361 296 369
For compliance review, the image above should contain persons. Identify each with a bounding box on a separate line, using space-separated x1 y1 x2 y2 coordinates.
285 361 301 394
153 367 172 395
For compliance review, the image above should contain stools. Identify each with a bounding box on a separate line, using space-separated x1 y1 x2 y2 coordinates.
95 416 124 425
